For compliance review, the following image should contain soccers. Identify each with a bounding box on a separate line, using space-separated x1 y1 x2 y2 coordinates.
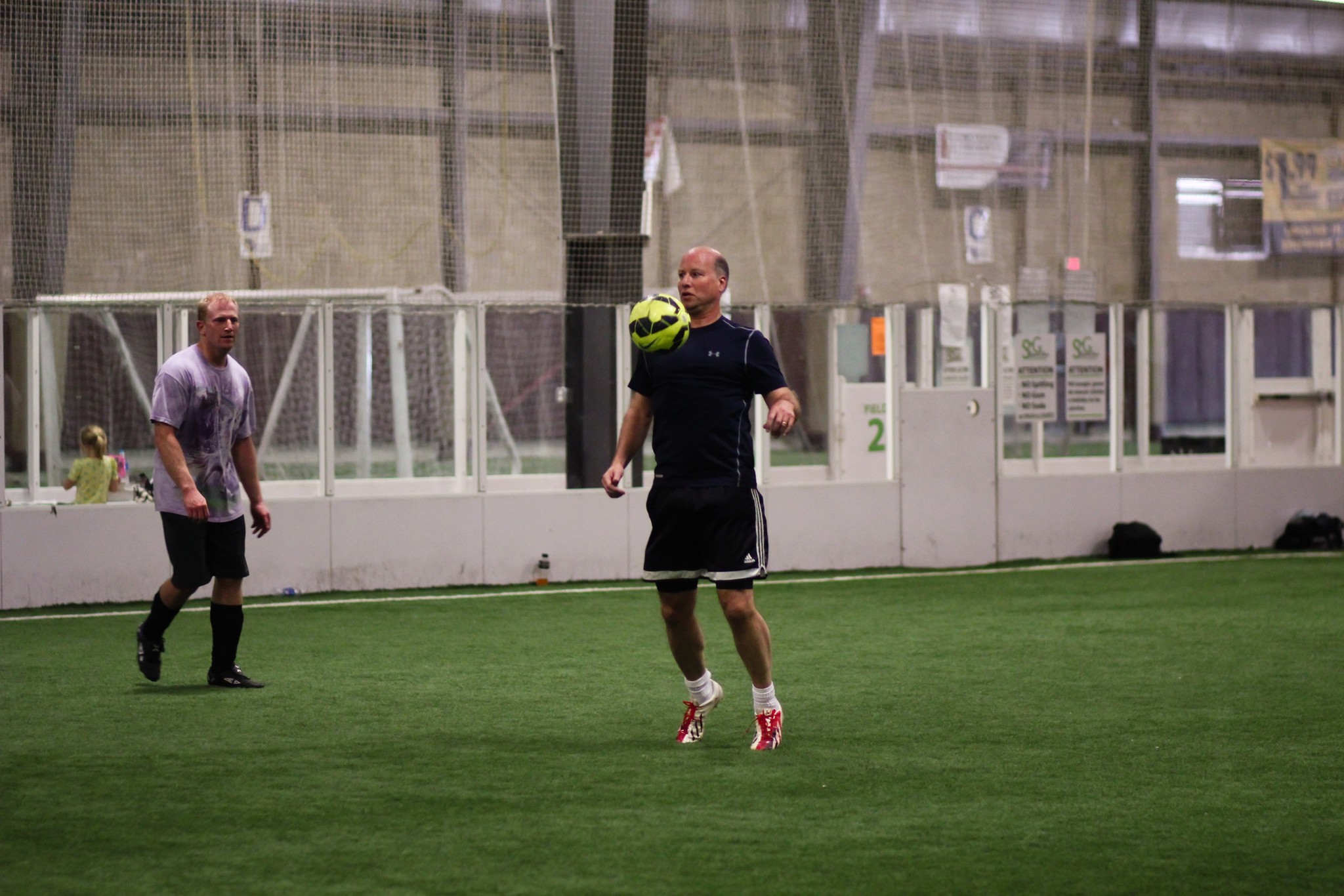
629 294 691 354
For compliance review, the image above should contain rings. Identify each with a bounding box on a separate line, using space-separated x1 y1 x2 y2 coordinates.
781 422 788 426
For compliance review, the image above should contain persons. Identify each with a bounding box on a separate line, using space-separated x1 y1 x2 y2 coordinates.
61 425 120 505
601 246 801 753
131 293 272 689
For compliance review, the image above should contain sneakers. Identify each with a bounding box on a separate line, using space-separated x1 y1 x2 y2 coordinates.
136 625 170 683
207 668 263 688
745 707 787 750
676 680 723 743
